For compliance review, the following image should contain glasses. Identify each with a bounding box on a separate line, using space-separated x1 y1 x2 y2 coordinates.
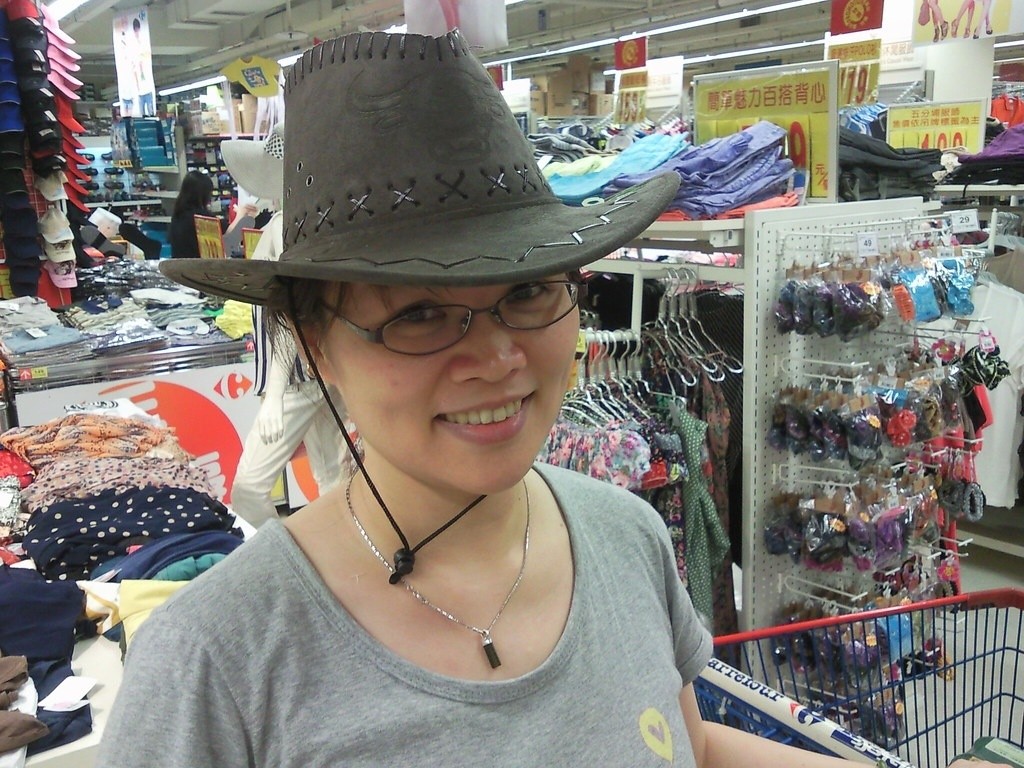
312 271 589 357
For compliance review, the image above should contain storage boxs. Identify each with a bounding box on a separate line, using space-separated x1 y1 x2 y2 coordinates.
567 54 590 94
140 156 168 167
130 119 157 129
590 61 610 93
132 146 165 158
208 104 242 134
571 91 589 116
234 94 266 133
159 244 173 258
136 223 167 230
534 70 573 94
589 92 614 116
142 229 170 243
179 110 202 137
546 94 572 117
530 91 545 116
132 128 157 138
134 137 158 146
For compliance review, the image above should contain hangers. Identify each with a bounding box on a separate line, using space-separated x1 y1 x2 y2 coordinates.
586 327 613 423
613 329 650 411
625 328 687 412
667 267 718 373
641 268 697 387
679 266 743 373
680 269 725 382
960 211 1024 251
595 330 639 426
603 329 652 421
560 324 609 428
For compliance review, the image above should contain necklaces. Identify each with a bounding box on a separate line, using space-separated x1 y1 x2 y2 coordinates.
345 467 531 669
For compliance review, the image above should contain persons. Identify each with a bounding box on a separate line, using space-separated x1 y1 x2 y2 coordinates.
167 171 223 258
219 121 352 526
99 34 878 768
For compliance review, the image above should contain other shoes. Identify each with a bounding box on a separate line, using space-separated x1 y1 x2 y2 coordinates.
78 152 161 201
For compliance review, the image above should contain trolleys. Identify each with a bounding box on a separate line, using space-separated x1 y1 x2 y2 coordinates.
686 585 1024 767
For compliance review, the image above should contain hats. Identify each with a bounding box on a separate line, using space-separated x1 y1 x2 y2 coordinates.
220 123 286 202
0 1 89 298
158 30 682 307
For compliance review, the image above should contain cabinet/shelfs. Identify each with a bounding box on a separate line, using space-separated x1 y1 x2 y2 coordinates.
126 125 192 222
